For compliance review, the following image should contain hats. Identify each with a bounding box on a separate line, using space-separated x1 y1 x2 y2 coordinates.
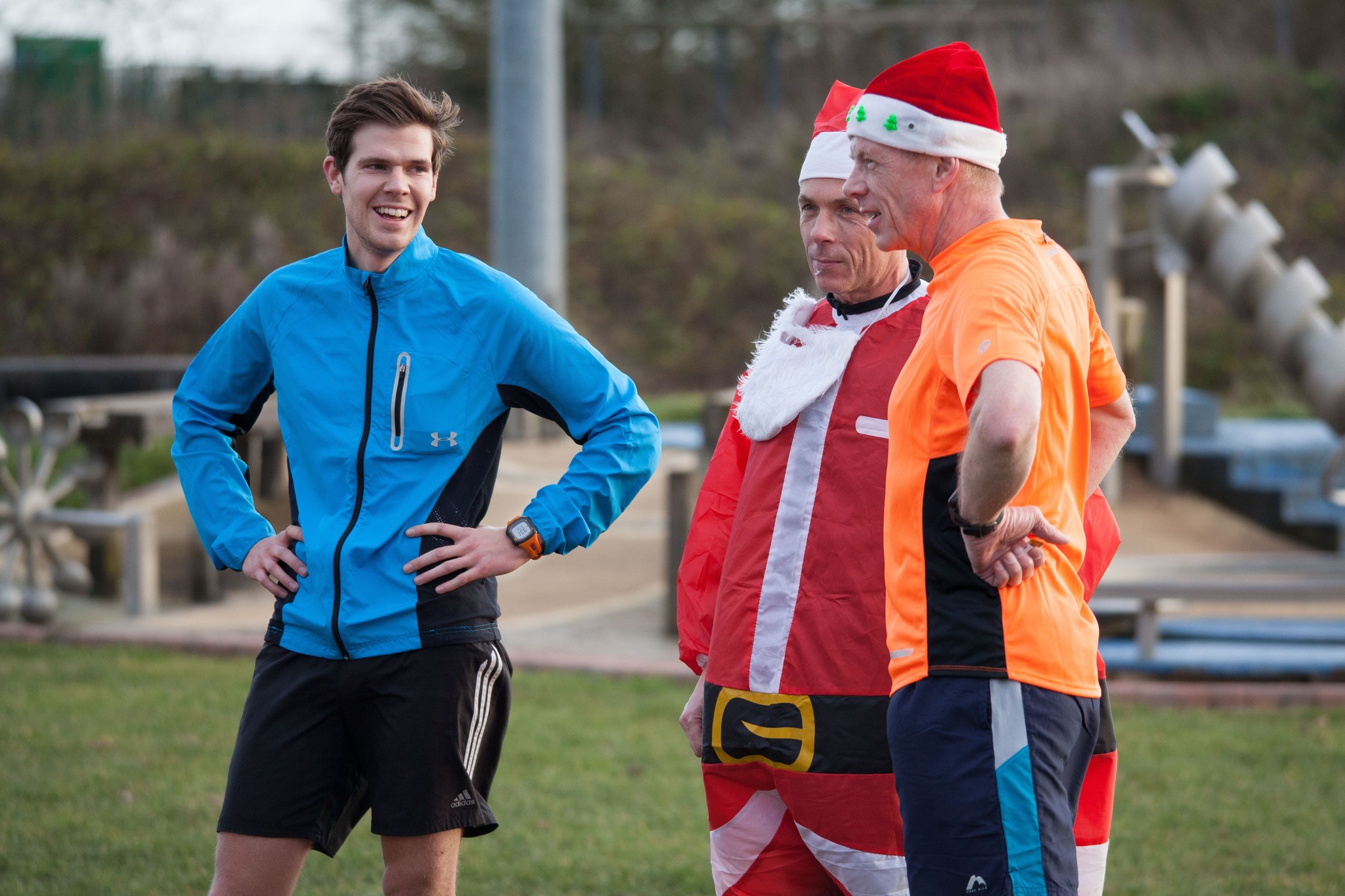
845 41 1008 173
799 80 867 180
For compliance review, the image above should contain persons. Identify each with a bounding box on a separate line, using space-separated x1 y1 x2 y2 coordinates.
841 43 1137 896
169 79 662 896
677 78 1122 896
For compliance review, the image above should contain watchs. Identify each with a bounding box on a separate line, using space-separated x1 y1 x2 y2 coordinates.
945 485 1003 538
505 514 544 561
